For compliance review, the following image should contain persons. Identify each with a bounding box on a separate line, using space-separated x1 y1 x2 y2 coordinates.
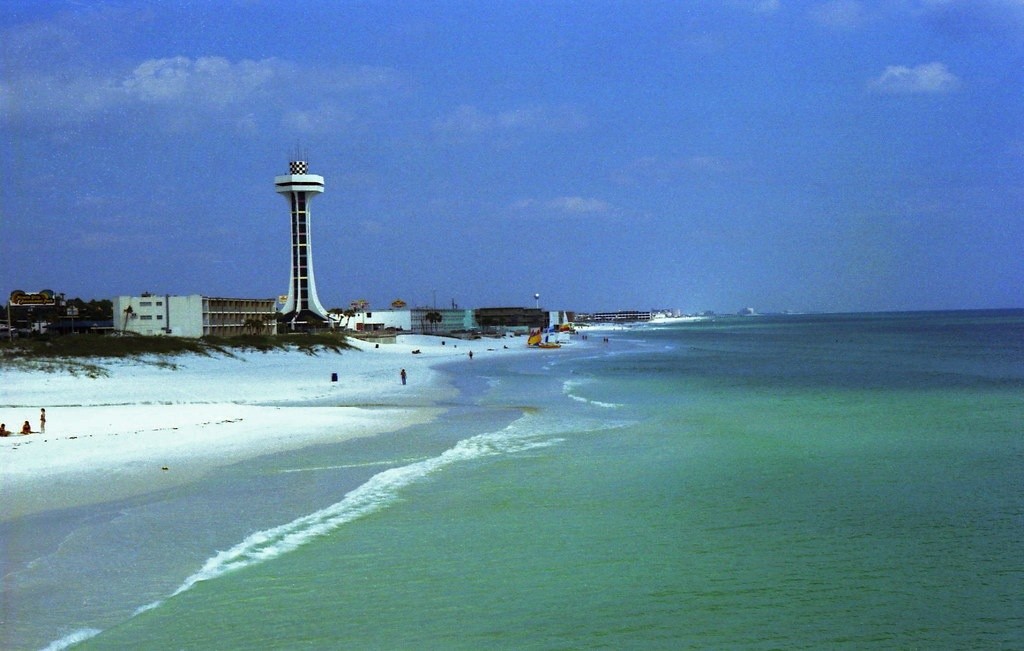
401 322 609 387
0 424 11 437
20 421 31 435
40 408 46 434
